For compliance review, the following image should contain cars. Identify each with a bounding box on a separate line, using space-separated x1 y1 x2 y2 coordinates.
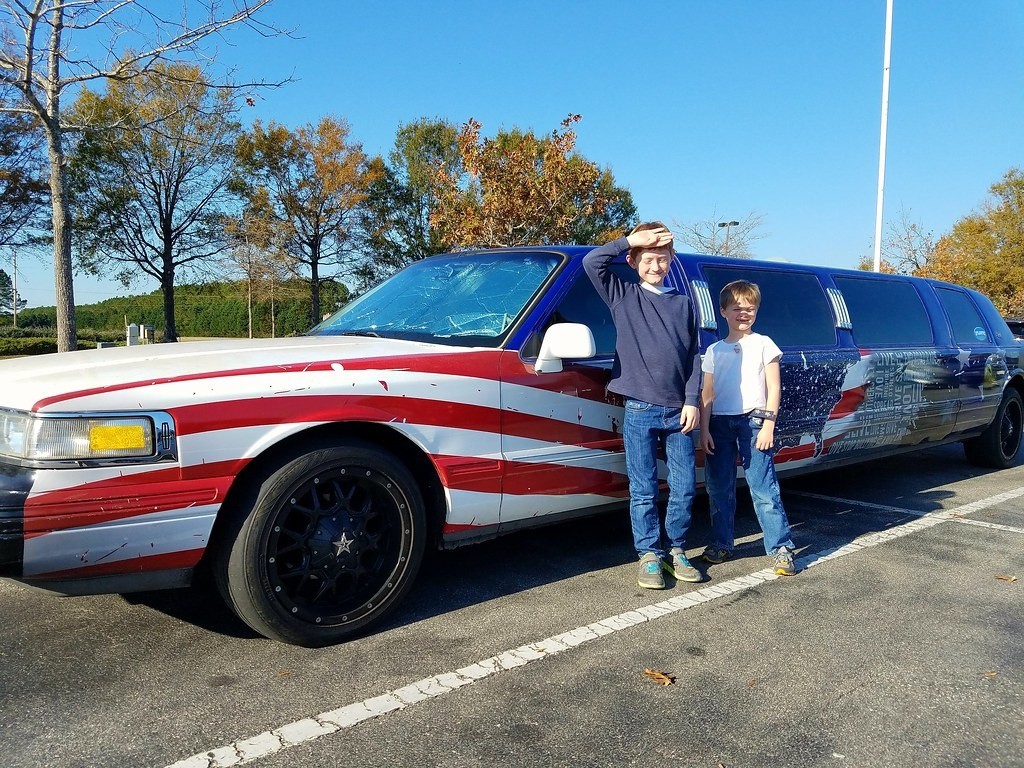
0 245 1023 645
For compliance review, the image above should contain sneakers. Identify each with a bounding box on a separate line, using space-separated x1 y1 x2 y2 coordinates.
772 545 797 576
703 544 734 564
638 547 704 589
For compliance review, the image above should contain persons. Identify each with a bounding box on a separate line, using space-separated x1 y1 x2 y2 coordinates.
581 220 704 590
699 280 797 576
